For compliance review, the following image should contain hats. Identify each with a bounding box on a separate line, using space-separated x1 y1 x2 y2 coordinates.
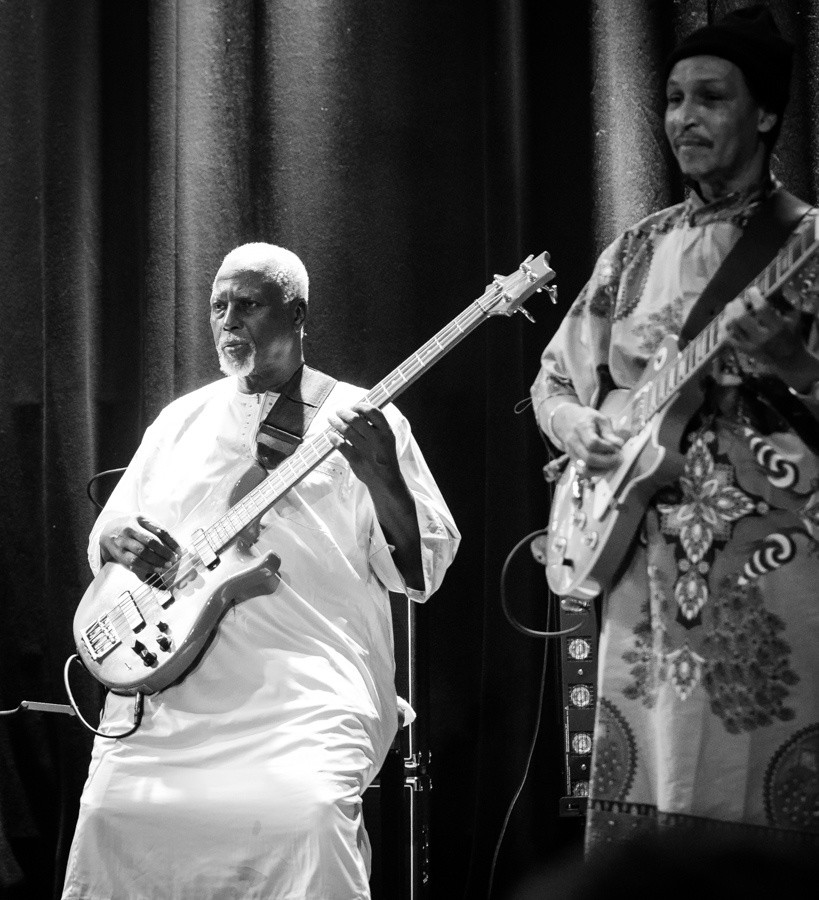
672 6 794 114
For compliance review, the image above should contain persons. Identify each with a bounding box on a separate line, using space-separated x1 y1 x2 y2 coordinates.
64 240 462 900
527 1 818 856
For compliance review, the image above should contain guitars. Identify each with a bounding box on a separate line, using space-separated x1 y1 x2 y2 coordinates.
72 249 559 695
546 211 819 603
